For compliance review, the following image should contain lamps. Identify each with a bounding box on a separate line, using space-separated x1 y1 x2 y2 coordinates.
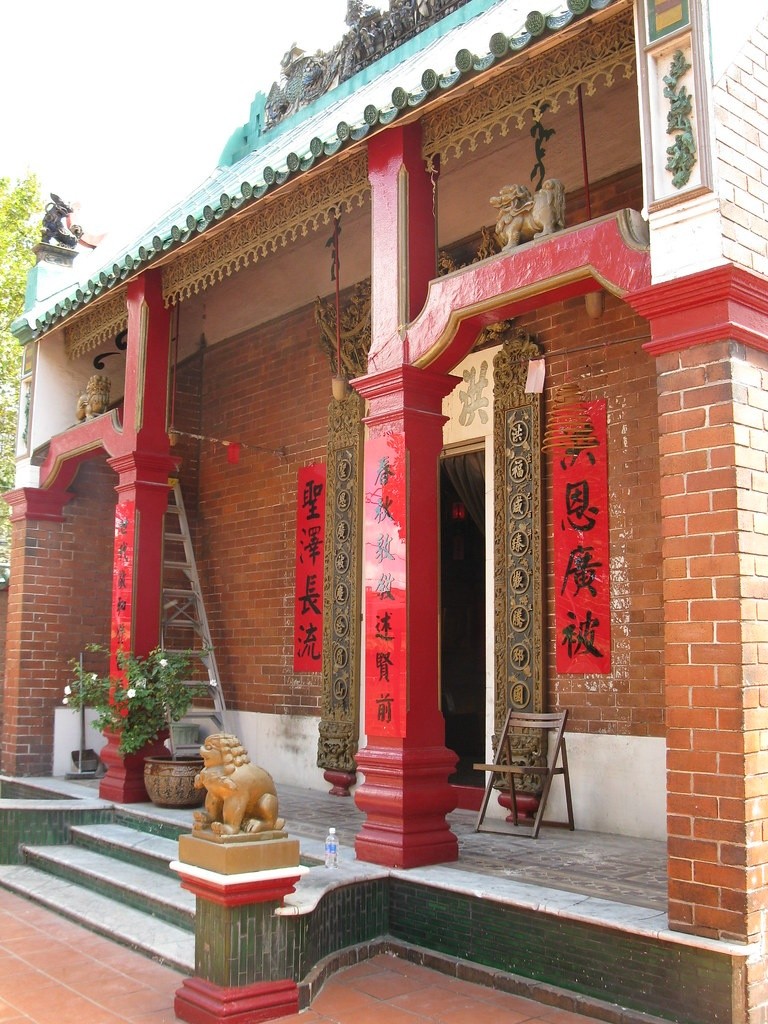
330 210 350 405
167 298 179 447
578 82 606 318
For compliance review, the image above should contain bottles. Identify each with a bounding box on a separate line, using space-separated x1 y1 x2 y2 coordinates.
324 827 339 869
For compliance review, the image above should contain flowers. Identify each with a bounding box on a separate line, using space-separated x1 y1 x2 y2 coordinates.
60 640 217 760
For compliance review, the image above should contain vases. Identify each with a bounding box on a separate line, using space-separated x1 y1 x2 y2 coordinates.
143 755 208 808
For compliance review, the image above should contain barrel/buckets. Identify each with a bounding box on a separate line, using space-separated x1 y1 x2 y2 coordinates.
168 722 200 748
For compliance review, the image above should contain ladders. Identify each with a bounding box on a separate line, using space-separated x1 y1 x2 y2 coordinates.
160 476 230 762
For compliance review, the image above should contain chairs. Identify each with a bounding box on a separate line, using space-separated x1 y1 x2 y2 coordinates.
472 709 574 840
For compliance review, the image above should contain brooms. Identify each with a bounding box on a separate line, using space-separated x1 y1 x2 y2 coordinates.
65 703 98 780
